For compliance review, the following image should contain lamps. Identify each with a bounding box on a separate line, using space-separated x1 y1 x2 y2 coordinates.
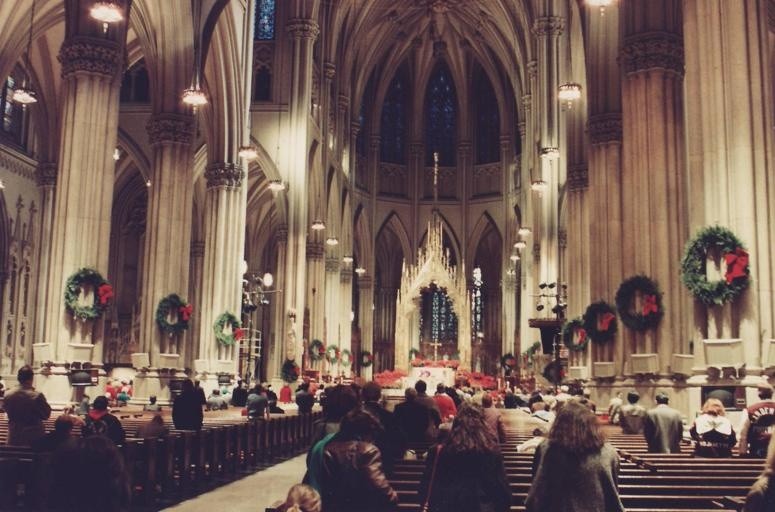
90 2 123 24
236 2 255 160
263 2 365 287
182 3 208 114
11 2 38 109
505 2 584 287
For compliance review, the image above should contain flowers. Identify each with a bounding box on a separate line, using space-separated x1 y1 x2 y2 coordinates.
156 293 193 336
681 227 752 306
308 340 542 389
562 320 590 351
278 359 300 385
613 274 666 331
542 359 565 383
62 268 113 320
213 312 242 346
582 301 618 343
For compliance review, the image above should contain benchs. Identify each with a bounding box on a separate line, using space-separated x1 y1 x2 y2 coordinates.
0 391 774 511
